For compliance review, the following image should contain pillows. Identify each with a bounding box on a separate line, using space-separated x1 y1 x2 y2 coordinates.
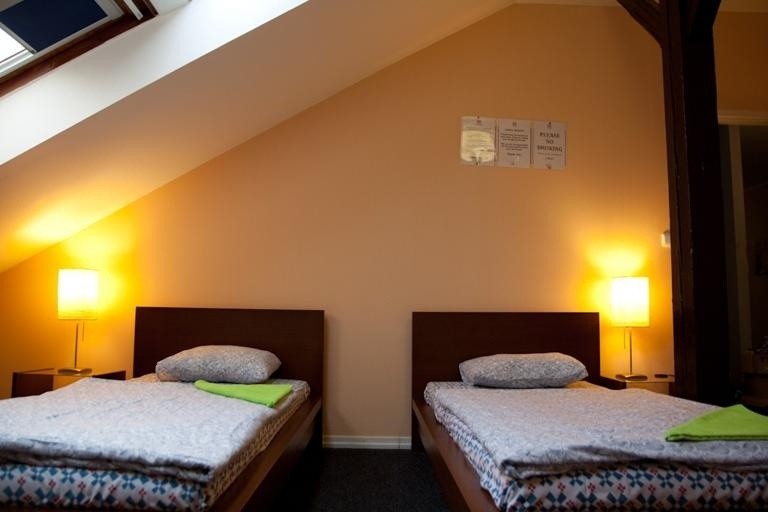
458 352 589 389
155 345 282 384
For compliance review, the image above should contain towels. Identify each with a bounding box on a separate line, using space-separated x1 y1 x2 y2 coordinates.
664 403 768 442
194 379 294 408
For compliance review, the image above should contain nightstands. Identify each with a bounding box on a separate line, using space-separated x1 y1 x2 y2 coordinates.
610 377 675 395
10 367 126 398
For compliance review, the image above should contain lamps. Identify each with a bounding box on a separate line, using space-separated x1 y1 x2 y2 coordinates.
609 276 650 380
57 268 99 374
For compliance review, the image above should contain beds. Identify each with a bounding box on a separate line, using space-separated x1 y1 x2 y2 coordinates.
411 311 768 512
0 306 325 512
